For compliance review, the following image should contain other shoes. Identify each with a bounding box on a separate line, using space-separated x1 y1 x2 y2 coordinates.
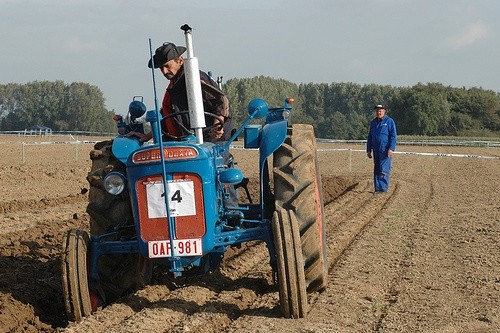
375 188 387 193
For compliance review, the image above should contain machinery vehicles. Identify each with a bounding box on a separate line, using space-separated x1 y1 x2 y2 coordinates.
59 23 326 322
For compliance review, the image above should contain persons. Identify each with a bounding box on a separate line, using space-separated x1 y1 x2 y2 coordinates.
147 42 229 142
366 103 397 194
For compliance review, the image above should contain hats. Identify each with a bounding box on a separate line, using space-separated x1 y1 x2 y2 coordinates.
374 103 386 110
148 42 186 68
